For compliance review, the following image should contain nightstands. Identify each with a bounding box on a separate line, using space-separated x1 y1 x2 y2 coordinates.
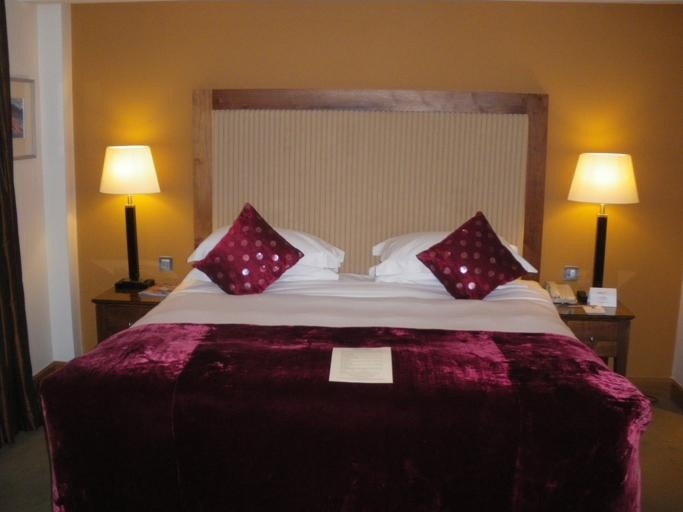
91 279 182 343
554 299 636 377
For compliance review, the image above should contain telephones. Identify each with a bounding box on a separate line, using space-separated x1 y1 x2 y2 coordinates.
545 281 577 304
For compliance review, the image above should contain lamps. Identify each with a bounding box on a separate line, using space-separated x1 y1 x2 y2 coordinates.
566 151 641 305
99 144 162 292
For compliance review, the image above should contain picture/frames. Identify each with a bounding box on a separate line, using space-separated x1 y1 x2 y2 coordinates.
8 76 38 162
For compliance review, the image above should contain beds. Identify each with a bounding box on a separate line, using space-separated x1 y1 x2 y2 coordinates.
39 87 652 511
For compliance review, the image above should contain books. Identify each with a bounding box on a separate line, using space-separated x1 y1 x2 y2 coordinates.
138 281 177 299
581 304 606 317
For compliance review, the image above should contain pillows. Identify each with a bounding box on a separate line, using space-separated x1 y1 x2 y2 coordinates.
196 204 304 298
188 224 344 285
370 228 537 291
415 211 527 302
